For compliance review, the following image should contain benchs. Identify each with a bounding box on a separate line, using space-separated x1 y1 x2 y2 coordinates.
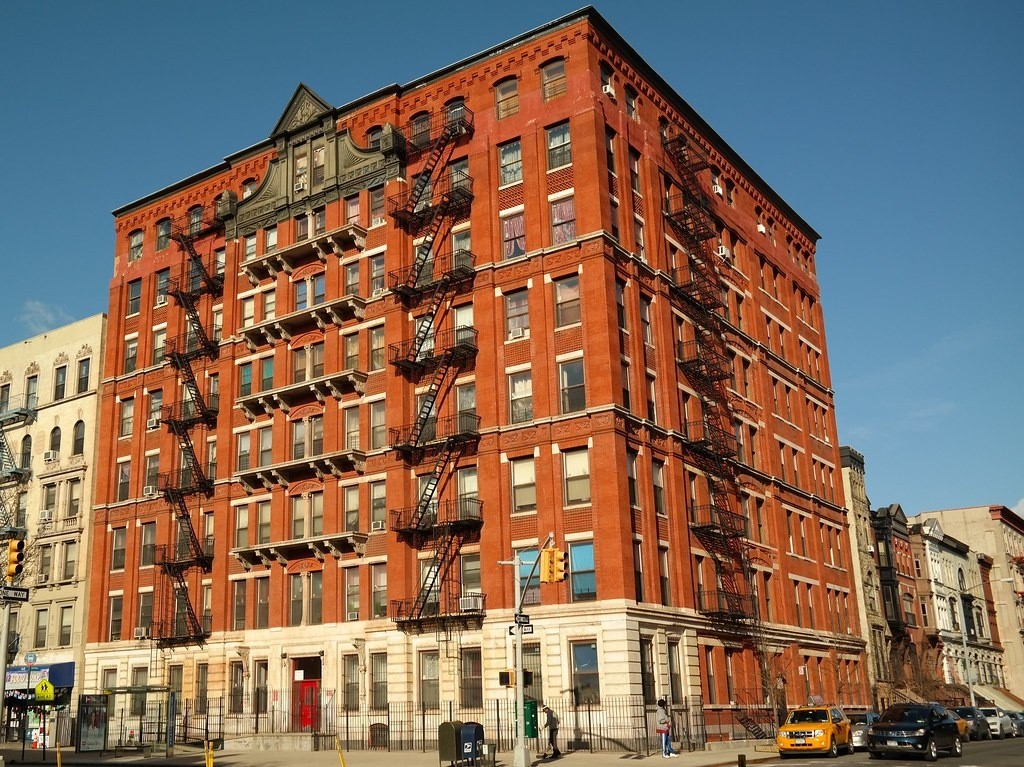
115 745 151 759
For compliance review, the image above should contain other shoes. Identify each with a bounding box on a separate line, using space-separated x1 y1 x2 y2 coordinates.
549 754 558 758
558 751 561 755
664 755 670 759
670 753 678 757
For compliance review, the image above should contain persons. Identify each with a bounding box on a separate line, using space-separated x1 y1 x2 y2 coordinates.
539 705 561 758
655 699 679 758
88 711 97 730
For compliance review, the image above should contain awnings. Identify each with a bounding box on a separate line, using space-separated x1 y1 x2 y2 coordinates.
6 661 75 690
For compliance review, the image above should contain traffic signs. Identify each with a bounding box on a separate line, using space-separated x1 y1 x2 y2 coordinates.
514 613 530 625
509 624 533 635
0 585 30 602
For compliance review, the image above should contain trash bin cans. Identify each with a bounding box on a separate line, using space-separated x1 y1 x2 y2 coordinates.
461 721 485 759
438 721 463 761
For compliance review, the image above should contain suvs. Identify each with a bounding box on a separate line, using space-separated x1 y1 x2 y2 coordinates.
866 703 963 762
775 702 854 758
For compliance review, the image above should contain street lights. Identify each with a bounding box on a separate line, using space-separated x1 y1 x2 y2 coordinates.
955 577 1016 706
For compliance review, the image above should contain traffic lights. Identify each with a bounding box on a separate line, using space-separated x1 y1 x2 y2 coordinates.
541 552 554 584
498 670 515 686
553 550 569 583
6 539 25 576
523 671 534 686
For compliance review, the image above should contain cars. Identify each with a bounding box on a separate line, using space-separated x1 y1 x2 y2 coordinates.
844 712 881 747
945 705 1024 743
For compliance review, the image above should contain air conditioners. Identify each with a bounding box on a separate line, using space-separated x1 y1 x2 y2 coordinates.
511 328 524 338
348 612 358 620
712 185 722 195
603 85 615 97
40 510 53 520
144 486 157 494
718 245 730 259
156 295 167 305
757 224 765 233
44 450 57 461
459 597 483 610
294 183 304 191
148 419 158 429
37 574 48 582
134 627 149 638
372 520 384 531
